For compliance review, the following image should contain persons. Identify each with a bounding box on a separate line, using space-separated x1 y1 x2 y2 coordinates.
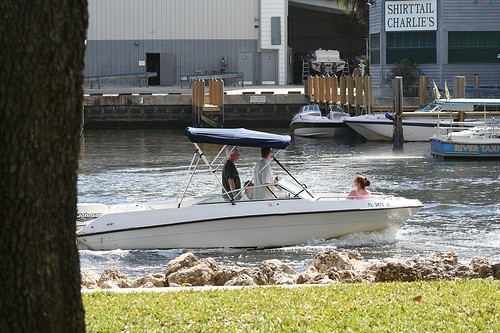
222 146 241 201
346 177 371 200
254 147 275 199
239 180 254 200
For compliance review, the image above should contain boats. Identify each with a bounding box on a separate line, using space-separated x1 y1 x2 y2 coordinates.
343 97 500 159
75 127 424 251
288 103 352 137
